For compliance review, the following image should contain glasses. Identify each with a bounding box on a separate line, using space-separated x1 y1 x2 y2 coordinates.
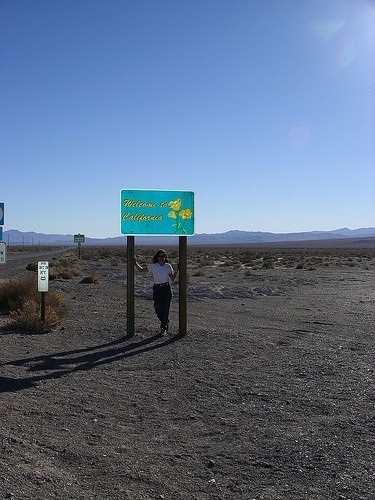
158 255 166 258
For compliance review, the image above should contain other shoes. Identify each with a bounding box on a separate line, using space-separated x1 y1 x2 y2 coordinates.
159 328 167 337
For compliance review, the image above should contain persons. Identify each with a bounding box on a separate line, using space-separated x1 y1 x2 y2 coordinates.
134 249 179 336
